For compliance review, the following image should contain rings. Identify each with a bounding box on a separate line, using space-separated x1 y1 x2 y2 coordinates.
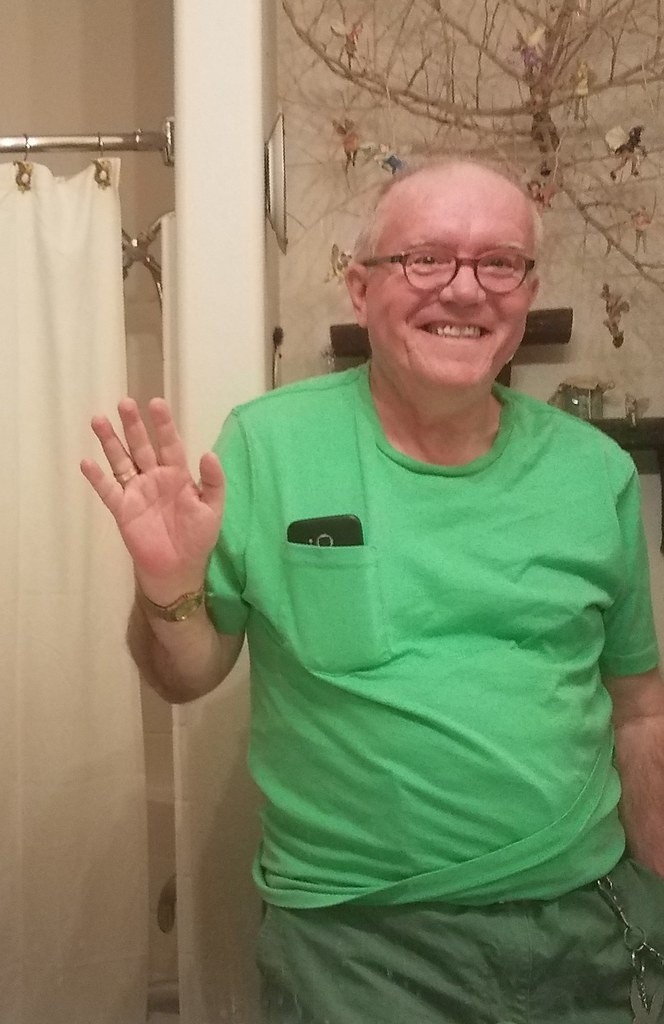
115 466 138 485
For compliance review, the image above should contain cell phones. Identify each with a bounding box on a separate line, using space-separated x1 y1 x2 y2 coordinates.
287 515 364 547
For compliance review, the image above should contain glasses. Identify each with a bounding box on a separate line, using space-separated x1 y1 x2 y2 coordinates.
360 243 535 295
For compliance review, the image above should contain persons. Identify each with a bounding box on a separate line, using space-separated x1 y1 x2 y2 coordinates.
82 158 664 1024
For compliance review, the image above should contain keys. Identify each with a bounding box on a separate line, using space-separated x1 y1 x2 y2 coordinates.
596 877 664 1012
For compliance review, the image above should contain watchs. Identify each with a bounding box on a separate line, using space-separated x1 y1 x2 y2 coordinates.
134 584 207 625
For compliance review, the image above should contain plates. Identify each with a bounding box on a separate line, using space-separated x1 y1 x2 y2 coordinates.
265 114 287 256
